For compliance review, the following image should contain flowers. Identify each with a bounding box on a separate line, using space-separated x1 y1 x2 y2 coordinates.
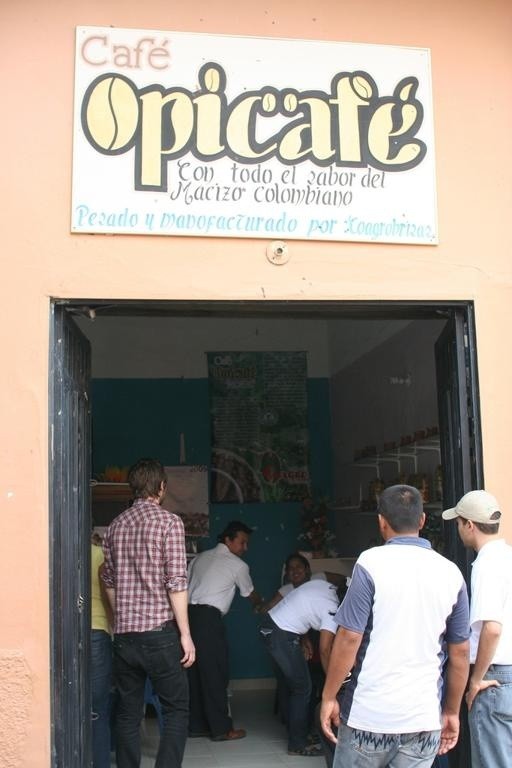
297 498 336 550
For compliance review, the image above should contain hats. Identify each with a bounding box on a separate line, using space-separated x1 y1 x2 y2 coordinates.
442 490 502 524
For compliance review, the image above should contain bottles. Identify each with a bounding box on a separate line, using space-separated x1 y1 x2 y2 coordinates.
367 467 443 508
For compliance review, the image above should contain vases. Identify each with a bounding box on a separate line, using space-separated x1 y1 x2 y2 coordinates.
311 549 326 560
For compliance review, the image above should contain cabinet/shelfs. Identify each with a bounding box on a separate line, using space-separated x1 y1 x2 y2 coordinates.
341 432 443 520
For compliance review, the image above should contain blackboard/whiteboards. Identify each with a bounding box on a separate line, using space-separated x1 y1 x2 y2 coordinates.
203 350 313 502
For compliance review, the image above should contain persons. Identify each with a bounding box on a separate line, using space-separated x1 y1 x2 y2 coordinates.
185 520 262 741
89 526 115 768
256 487 469 766
442 490 512 768
99 457 196 768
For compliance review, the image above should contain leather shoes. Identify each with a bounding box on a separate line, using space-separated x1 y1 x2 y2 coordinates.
212 728 246 740
288 745 324 755
307 733 322 744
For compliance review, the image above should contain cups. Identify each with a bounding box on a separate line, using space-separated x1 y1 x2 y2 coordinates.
380 427 438 450
